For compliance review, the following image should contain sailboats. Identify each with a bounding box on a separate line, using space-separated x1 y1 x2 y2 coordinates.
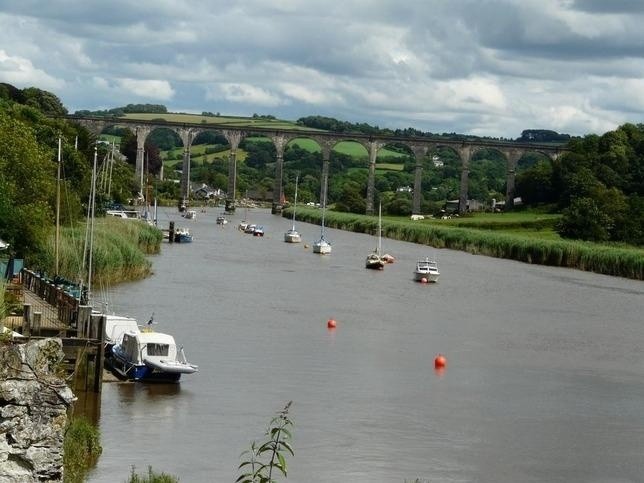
364 199 386 271
51 138 199 385
283 175 302 245
312 175 333 255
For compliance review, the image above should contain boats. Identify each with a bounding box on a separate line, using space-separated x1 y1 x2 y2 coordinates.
214 217 266 238
223 199 235 215
412 257 440 284
